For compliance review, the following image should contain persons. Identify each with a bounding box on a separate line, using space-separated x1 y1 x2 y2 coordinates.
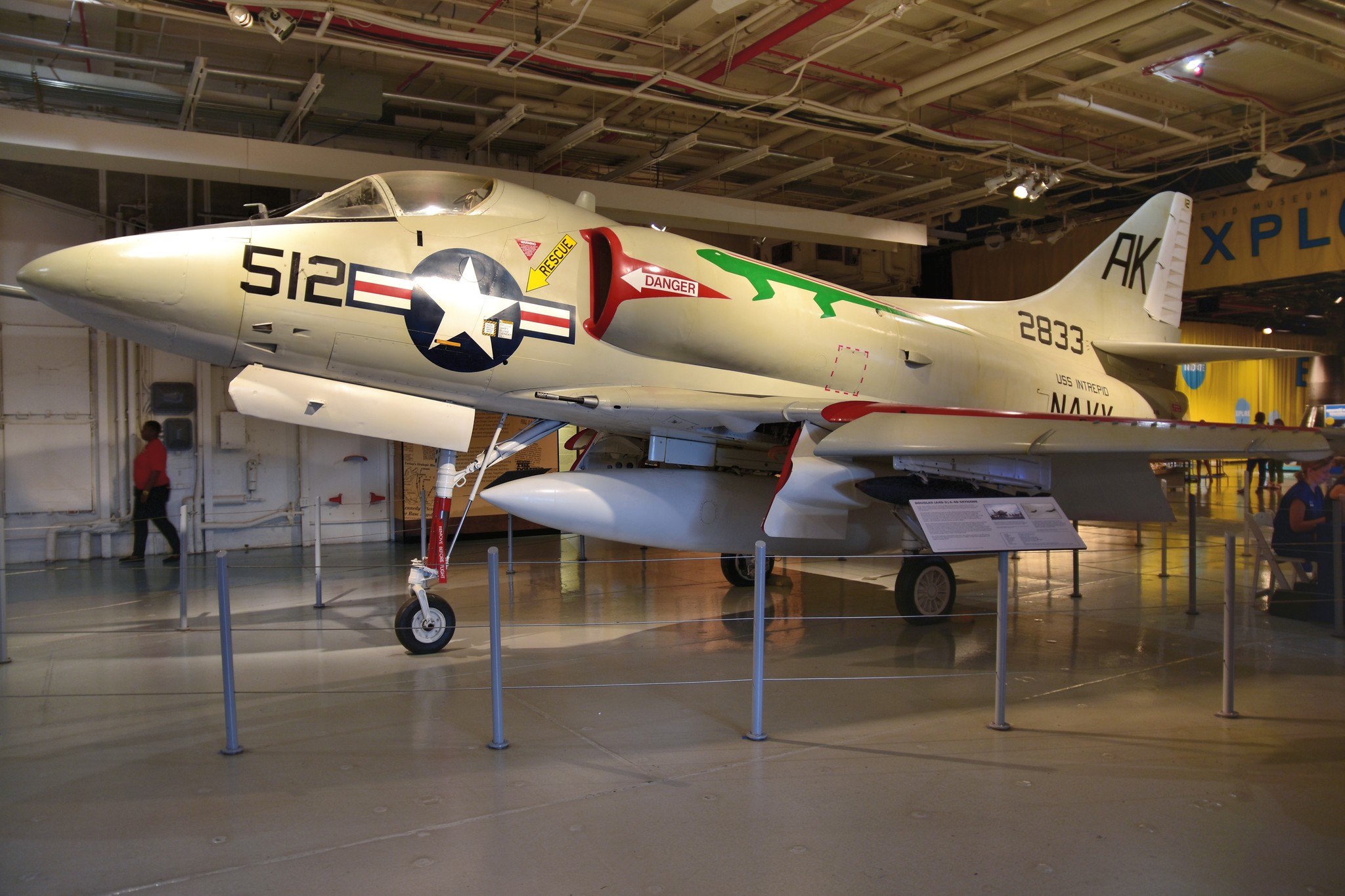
1195 419 1214 483
1269 456 1335 597
1266 418 1286 490
118 420 181 564
1236 411 1268 493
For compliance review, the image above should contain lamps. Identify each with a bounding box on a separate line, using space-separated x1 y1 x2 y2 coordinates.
983 162 1063 203
225 3 253 28
259 7 298 45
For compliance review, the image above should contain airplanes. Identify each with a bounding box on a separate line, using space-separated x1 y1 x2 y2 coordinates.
16 167 1345 656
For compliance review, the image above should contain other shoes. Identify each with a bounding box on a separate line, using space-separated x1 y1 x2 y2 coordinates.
1256 488 1263 492
120 555 144 563
1272 485 1281 490
163 556 180 563
1238 489 1244 492
1265 483 1274 488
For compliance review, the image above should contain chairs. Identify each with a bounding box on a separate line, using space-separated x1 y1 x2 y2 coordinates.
1244 509 1309 602
